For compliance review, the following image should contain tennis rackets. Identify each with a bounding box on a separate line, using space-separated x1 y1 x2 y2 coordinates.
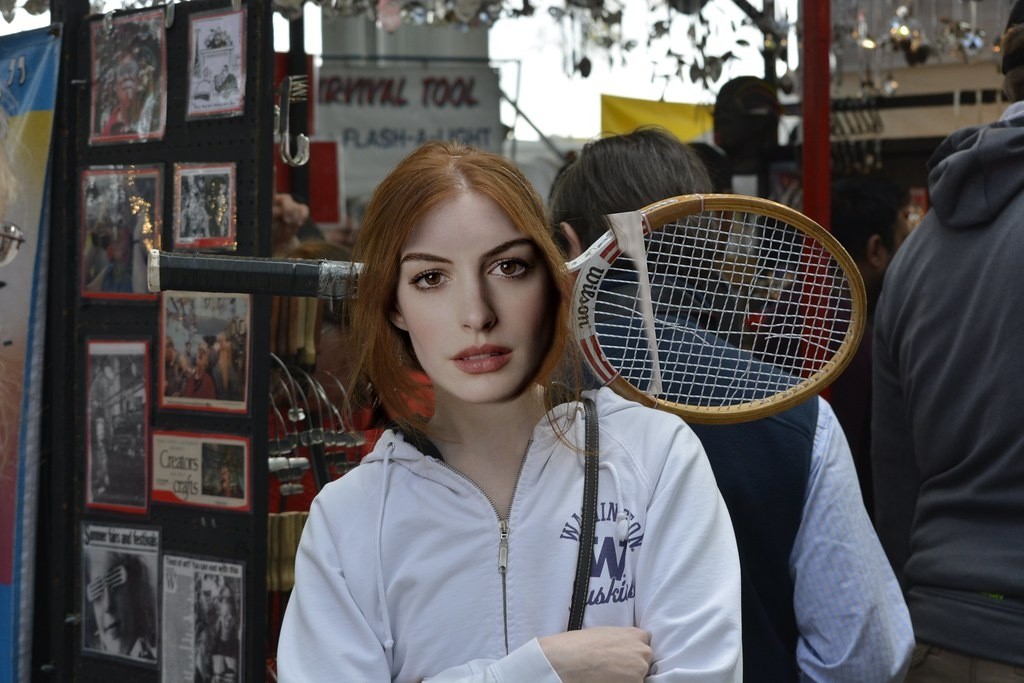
146 192 869 424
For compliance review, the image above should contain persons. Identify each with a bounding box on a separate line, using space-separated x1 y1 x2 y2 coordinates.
276 142 743 683
870 1 1023 683
85 24 245 682
536 126 914 683
689 142 909 530
